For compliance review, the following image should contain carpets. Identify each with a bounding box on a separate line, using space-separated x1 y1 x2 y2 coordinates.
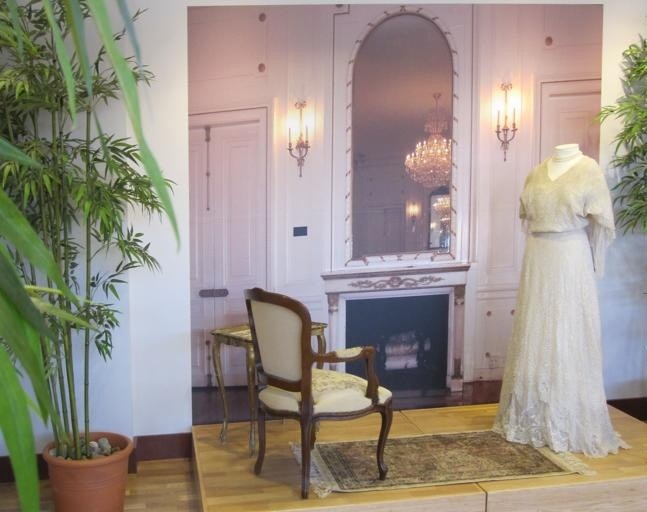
288 430 597 499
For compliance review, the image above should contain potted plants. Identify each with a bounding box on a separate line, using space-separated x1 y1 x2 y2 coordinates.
1 1 182 512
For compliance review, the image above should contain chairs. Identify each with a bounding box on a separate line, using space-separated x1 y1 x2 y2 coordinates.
244 288 394 500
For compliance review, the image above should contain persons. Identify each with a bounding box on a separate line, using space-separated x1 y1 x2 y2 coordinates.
491 144 632 457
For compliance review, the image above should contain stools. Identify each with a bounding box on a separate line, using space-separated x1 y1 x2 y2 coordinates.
209 323 327 459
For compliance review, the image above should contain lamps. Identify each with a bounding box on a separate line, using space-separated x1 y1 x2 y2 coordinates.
404 95 451 188
433 195 451 220
493 82 521 162
405 199 423 232
287 99 314 177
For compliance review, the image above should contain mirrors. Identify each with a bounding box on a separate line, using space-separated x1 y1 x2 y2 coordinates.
344 6 458 267
